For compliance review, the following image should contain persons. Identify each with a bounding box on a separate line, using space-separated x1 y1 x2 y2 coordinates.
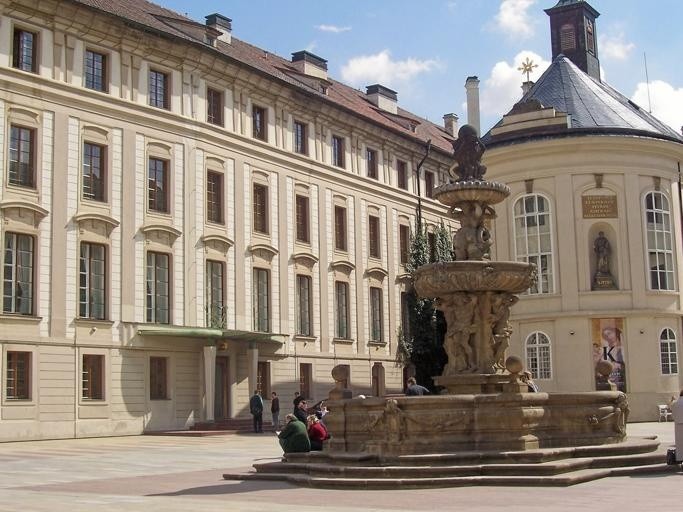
593 327 625 380
452 124 487 181
667 389 683 463
431 290 520 374
405 376 430 396
446 199 483 261
249 390 329 452
593 230 613 276
521 370 539 392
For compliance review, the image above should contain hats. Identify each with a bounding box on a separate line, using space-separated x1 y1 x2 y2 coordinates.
293 396 304 404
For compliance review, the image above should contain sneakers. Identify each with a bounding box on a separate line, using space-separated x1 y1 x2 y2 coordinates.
254 430 263 433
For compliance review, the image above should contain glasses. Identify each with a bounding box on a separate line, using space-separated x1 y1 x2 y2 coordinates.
302 401 308 404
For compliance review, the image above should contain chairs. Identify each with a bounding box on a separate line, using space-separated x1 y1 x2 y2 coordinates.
658 405 672 422
